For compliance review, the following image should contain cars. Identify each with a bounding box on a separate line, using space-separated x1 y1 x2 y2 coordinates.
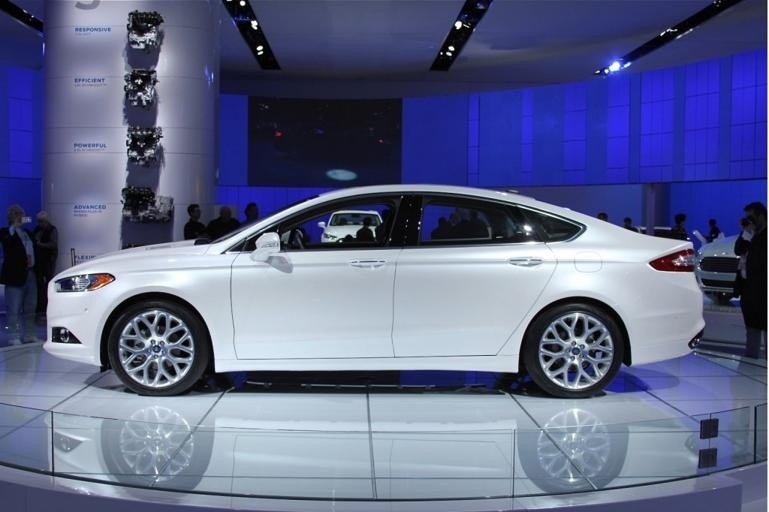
42 184 705 399
697 234 745 305
41 374 716 511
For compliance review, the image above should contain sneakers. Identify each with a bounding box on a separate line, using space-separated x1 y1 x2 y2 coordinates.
8 336 38 345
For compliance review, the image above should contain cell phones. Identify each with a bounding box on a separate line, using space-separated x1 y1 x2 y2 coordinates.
21 217 32 223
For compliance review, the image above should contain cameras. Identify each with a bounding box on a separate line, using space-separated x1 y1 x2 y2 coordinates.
741 215 756 227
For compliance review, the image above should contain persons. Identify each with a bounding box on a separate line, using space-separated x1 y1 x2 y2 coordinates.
0 204 58 345
184 202 258 239
356 217 374 241
430 216 449 239
622 201 767 360
